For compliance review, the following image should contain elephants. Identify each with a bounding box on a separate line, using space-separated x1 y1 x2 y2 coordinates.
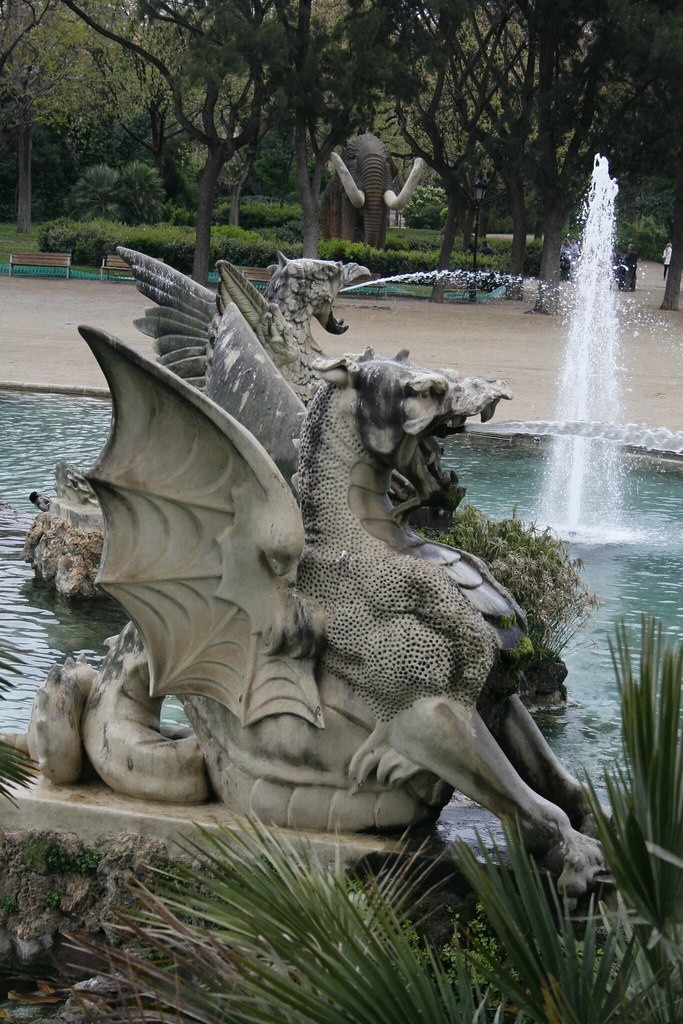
320 133 423 250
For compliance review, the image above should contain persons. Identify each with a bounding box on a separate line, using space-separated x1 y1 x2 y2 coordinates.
662 243 673 280
560 232 640 292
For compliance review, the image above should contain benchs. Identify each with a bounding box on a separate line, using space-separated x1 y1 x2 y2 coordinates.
100 254 166 283
7 250 73 279
437 275 480 303
231 265 273 284
339 272 386 300
